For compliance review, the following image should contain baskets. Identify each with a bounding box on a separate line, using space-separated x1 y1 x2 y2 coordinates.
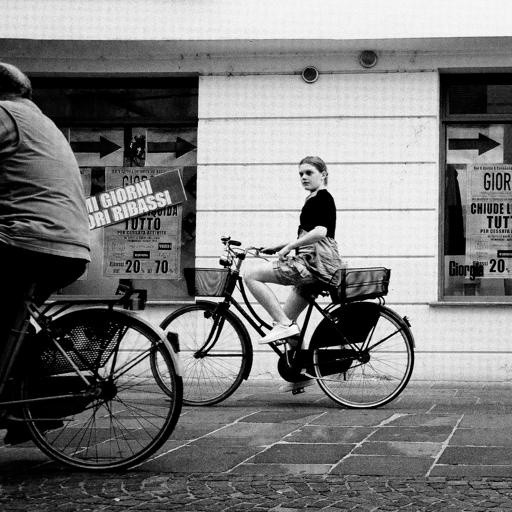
183 267 229 296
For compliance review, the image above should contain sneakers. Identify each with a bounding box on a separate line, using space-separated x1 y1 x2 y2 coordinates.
279 378 316 393
258 319 301 345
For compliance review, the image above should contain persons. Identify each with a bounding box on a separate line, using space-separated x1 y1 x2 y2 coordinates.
242 155 337 392
0 60 92 446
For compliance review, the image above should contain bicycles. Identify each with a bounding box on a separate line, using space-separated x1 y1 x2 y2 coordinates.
149 234 416 413
0 268 185 476
